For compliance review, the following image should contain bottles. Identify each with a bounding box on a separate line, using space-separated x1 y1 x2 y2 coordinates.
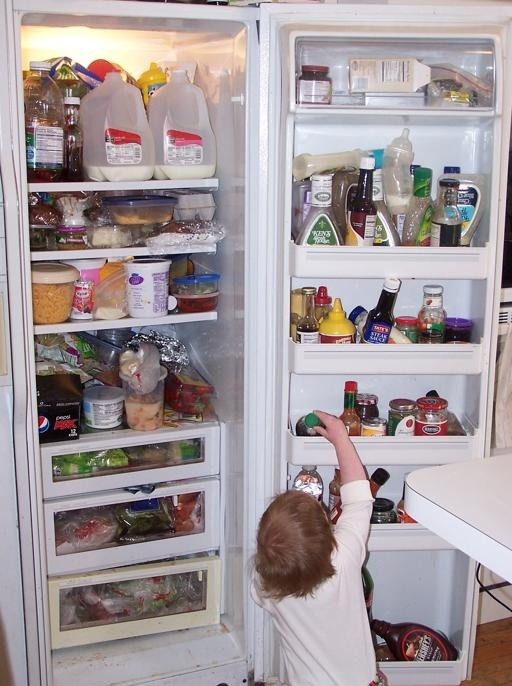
32 264 80 324
293 128 484 248
119 365 167 431
295 66 333 106
361 555 459 661
297 379 466 437
55 223 89 248
139 60 168 106
291 278 474 346
292 466 416 523
23 60 82 180
83 387 125 429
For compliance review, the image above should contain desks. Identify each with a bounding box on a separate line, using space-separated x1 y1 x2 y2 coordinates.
404 453 512 585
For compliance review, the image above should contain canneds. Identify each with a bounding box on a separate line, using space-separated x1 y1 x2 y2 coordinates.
444 317 474 343
416 397 449 436
396 316 418 343
387 398 416 436
373 498 397 524
356 393 379 419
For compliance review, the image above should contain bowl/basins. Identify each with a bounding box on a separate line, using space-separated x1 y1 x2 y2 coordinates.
105 194 177 225
173 273 220 313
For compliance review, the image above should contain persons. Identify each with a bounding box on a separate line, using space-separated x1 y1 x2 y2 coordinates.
250 408 385 686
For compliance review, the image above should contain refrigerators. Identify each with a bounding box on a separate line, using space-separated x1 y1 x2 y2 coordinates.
0 1 510 686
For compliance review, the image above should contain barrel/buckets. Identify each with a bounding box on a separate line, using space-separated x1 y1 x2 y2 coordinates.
148 68 217 181
21 60 65 182
81 70 153 182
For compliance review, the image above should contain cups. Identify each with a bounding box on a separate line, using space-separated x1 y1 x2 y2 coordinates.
62 258 108 320
